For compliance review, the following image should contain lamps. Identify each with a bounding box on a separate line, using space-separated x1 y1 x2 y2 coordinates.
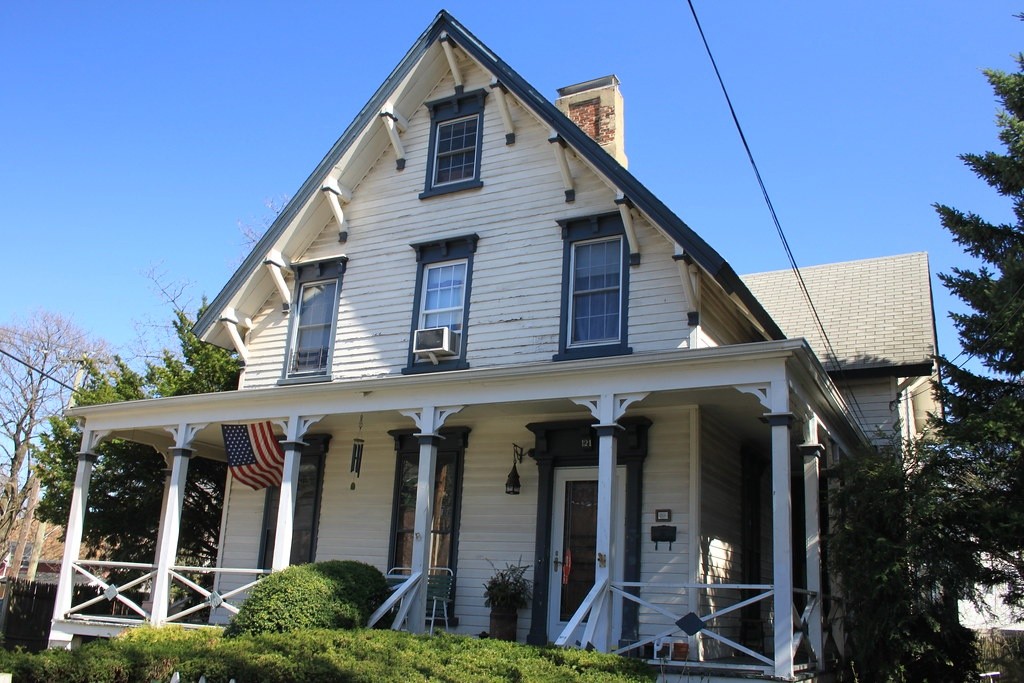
504 443 534 494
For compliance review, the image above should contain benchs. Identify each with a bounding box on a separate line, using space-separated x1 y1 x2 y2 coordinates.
380 564 453 638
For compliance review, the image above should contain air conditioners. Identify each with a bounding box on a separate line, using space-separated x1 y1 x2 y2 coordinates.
412 327 459 356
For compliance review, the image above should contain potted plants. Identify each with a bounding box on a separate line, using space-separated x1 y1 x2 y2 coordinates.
483 550 535 641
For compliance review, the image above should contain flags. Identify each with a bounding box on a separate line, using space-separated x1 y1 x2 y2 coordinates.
221 420 285 493
